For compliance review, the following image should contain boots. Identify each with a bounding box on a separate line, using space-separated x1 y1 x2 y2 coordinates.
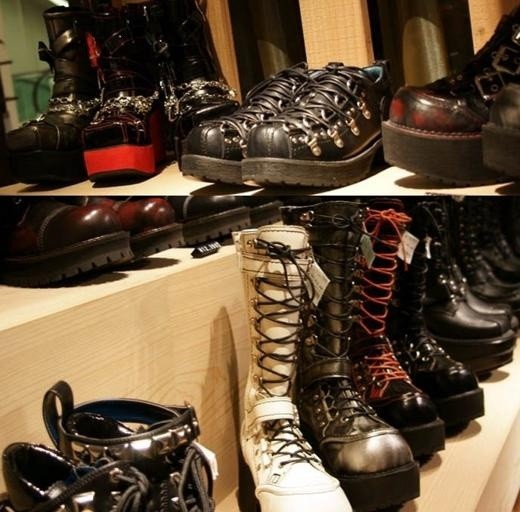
4 381 217 512
80 7 177 181
142 2 239 172
385 201 485 426
417 200 514 375
441 196 520 338
1 5 101 190
279 200 421 512
351 198 445 460
232 220 353 512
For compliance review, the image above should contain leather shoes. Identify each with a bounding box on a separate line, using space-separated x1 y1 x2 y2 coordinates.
381 7 520 186
0 194 284 285
183 60 393 187
